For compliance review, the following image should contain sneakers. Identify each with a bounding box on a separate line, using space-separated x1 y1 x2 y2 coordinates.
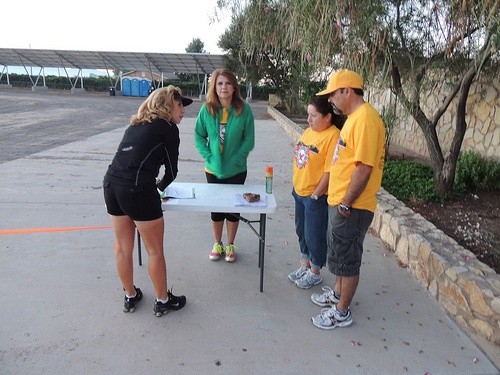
123 287 142 312
311 286 340 306
296 271 322 289
152 291 186 316
311 305 352 330
288 265 308 282
209 242 224 260
225 244 237 261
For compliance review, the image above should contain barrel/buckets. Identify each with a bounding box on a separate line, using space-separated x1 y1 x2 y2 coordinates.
110 88 115 96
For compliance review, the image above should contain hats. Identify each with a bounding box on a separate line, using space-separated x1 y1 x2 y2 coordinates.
315 69 363 96
173 91 193 106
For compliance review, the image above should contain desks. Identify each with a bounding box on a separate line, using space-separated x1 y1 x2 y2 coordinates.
138 183 278 294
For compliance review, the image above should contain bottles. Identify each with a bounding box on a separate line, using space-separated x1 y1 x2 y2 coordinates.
265 166 273 193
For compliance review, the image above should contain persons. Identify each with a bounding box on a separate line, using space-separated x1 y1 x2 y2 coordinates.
194 68 254 261
103 85 193 317
310 71 384 330
288 101 341 289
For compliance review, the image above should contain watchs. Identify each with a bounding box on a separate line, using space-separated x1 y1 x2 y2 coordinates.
312 193 318 200
340 202 351 212
